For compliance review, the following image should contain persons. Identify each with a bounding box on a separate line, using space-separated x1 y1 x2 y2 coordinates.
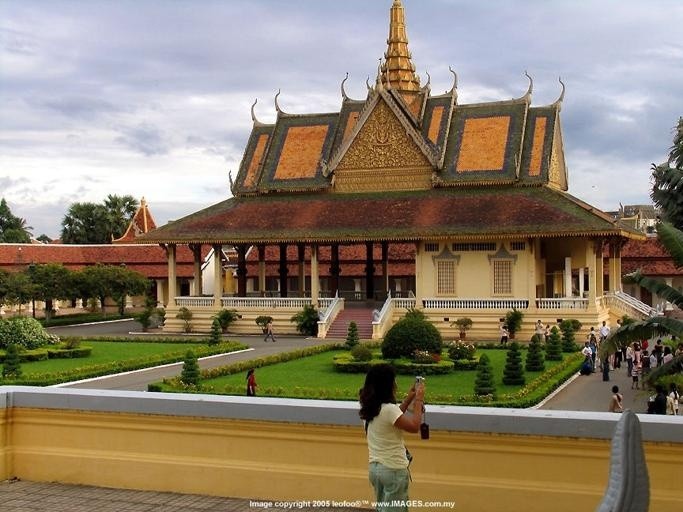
501 325 510 344
499 318 506 340
535 320 543 340
359 361 425 512
264 318 279 343
581 318 683 416
245 369 257 397
544 325 550 342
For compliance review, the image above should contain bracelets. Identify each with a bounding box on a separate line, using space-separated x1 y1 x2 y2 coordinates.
405 398 411 404
414 399 424 401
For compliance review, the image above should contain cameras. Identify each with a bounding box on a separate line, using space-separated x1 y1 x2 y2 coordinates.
416 376 425 384
